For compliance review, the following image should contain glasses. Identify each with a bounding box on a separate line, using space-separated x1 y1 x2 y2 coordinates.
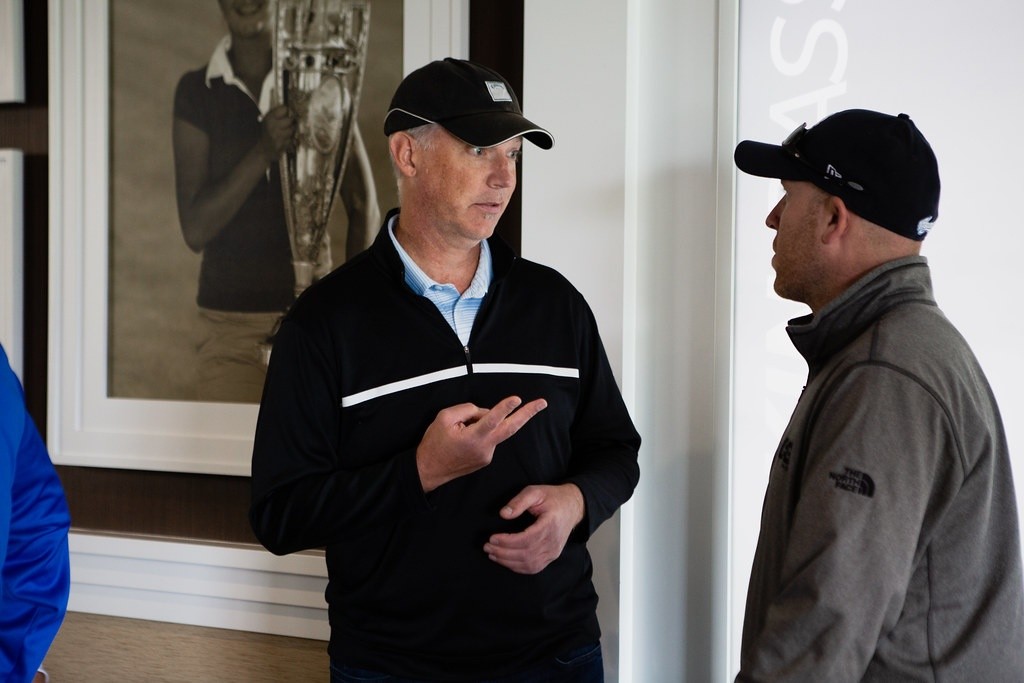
782 121 863 191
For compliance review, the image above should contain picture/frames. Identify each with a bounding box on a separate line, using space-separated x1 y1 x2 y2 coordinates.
48 0 469 476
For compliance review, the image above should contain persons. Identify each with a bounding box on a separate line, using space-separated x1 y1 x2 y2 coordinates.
0 345 71 683
250 58 643 683
736 110 1024 683
170 0 383 316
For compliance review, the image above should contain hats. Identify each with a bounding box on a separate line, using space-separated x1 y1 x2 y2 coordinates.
384 57 555 150
734 109 940 241
